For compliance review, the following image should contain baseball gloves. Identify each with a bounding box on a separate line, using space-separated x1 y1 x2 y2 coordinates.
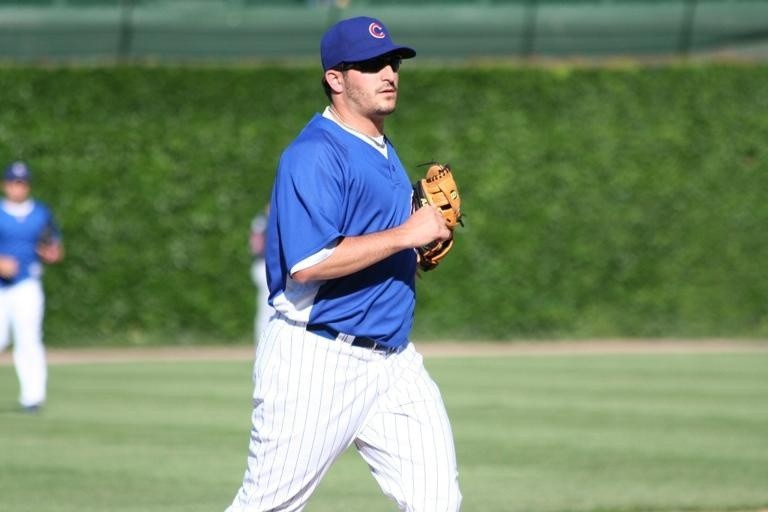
411 162 461 271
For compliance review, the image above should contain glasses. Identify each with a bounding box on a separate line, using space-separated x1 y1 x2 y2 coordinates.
342 54 401 73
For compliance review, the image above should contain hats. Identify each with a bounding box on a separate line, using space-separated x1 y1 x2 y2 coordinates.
320 16 416 71
1 159 31 181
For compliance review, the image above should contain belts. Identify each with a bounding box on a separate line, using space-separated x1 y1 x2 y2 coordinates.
306 323 397 353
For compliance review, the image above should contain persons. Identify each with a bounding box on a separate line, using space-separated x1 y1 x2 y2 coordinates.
224 16 464 512
0 158 65 412
249 202 274 408
